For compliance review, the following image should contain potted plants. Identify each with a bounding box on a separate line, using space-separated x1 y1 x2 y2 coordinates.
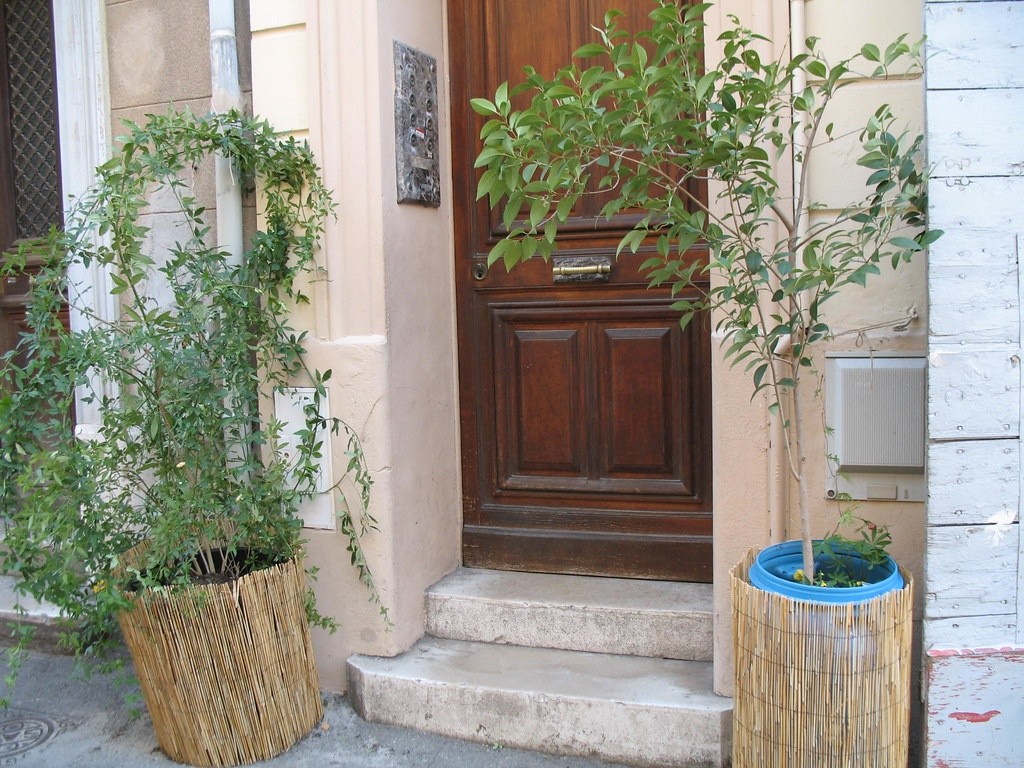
1 107 396 768
468 1 962 766
